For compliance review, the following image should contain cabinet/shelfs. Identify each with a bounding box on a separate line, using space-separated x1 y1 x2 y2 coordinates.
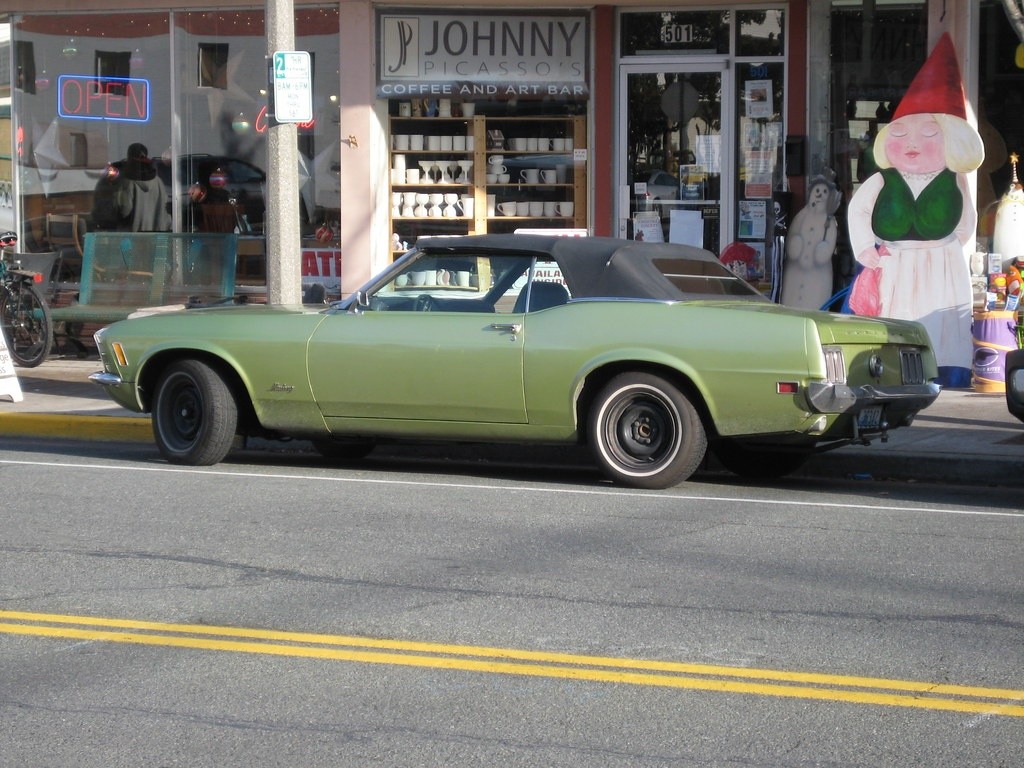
387 114 587 291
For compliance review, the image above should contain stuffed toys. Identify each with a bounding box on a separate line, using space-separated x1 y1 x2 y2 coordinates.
1007 265 1024 298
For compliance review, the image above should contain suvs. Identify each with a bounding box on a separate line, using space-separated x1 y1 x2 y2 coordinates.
91 153 266 232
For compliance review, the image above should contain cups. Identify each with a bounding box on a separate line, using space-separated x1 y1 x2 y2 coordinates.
485 138 573 218
390 97 476 288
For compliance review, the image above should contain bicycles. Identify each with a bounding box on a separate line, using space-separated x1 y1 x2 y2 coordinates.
0 227 54 368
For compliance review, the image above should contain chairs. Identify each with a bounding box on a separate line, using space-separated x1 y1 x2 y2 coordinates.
514 281 569 312
202 202 248 279
46 213 106 282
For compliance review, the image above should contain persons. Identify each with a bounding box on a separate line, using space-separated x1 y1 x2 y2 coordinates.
752 88 766 101
113 143 175 304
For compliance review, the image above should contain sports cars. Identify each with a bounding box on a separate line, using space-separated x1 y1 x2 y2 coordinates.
88 234 944 490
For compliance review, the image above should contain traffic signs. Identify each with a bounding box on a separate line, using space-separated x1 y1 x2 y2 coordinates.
273 50 313 123
